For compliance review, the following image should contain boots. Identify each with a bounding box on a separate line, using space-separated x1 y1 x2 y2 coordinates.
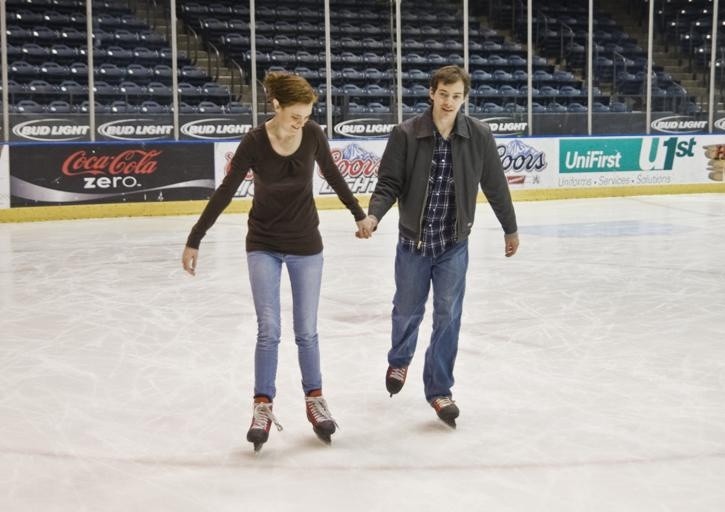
246 394 273 442
306 389 336 436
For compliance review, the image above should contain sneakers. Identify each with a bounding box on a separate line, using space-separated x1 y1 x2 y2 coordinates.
385 365 407 393
431 396 459 420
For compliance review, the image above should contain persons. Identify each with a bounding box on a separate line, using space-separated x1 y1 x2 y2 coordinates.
354 64 520 420
181 69 373 443
